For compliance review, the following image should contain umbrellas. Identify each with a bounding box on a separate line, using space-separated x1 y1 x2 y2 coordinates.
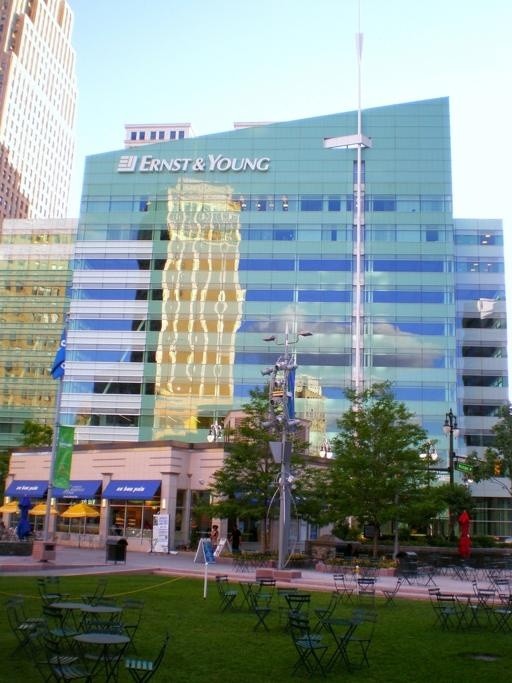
27 502 56 531
0 501 19 529
59 501 100 548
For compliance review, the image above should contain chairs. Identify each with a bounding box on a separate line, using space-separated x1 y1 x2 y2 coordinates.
403 551 512 636
2 574 171 683
209 557 402 682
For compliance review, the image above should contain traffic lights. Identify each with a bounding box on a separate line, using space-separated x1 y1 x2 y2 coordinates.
495 461 500 474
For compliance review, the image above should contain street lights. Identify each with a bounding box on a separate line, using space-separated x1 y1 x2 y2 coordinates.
207 422 223 444
262 321 314 570
318 442 332 458
443 409 462 538
419 439 439 489
455 460 473 474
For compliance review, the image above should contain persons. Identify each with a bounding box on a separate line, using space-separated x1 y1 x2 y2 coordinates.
229 524 242 551
211 524 219 551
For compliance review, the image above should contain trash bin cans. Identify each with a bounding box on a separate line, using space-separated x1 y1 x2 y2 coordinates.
106 537 128 566
396 551 417 574
